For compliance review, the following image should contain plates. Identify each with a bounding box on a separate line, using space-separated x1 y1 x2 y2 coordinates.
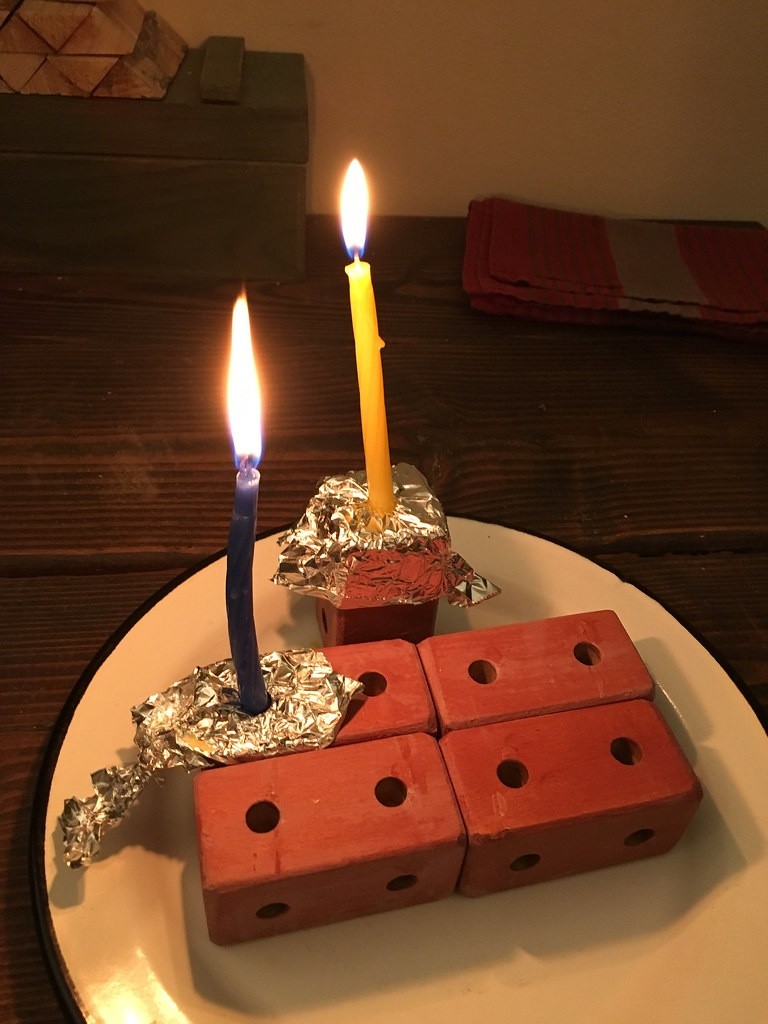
26 515 768 1024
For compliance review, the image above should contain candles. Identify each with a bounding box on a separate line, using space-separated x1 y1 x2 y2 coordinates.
218 283 270 717
336 155 397 518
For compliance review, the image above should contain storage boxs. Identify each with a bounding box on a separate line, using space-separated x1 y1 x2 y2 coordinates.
0 49 305 278
